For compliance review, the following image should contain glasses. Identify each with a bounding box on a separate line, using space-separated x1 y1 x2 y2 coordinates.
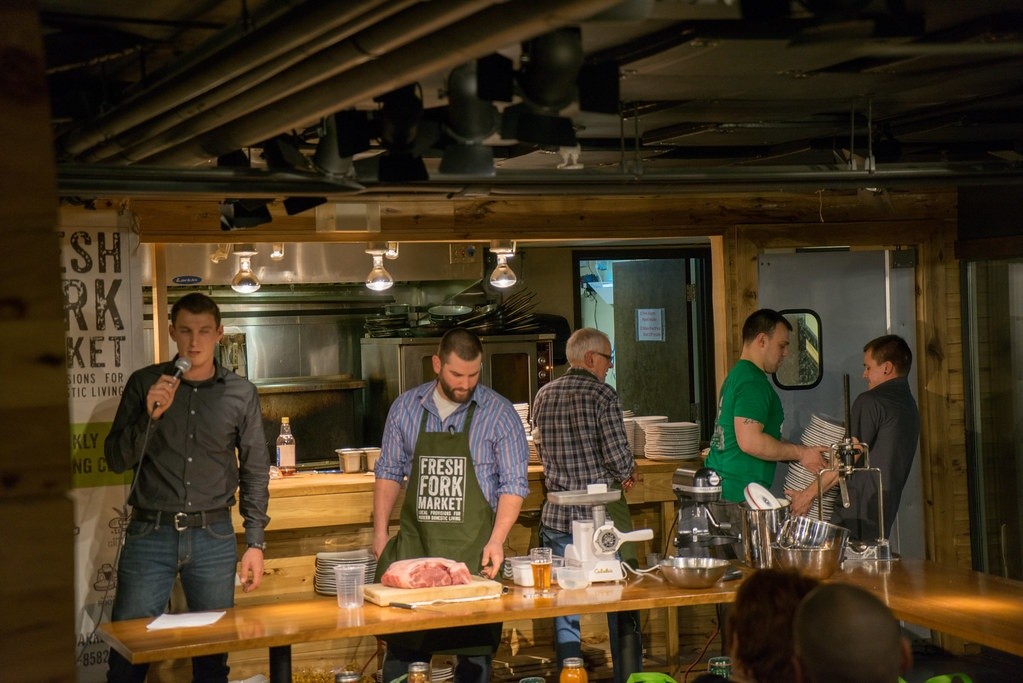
591 350 616 364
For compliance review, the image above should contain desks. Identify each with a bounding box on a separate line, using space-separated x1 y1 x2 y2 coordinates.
95 557 1023 683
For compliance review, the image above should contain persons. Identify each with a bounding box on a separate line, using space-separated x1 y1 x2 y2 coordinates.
726 566 914 683
104 290 272 683
783 334 920 547
370 327 530 683
530 326 643 673
703 308 833 505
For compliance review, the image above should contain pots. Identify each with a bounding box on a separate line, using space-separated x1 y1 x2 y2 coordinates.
364 278 540 338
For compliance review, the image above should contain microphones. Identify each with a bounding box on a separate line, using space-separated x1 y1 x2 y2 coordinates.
154 357 192 409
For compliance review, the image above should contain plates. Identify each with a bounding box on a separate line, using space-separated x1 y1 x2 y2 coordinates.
784 413 847 523
622 410 701 462
512 404 541 465
313 550 377 594
431 662 454 683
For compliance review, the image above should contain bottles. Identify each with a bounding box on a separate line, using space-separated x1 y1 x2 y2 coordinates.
560 658 588 683
407 662 431 683
276 416 295 475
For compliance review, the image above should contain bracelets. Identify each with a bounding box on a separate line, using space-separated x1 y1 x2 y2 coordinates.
246 542 267 552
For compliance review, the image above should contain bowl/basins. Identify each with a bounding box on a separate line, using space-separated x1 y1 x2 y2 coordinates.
558 567 588 588
656 556 732 589
739 498 850 580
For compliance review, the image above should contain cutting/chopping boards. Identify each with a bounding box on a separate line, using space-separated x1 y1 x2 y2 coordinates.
358 575 503 606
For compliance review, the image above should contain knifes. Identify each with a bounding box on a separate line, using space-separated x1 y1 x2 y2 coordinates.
390 602 443 613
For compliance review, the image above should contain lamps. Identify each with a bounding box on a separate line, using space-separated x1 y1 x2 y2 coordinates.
489 239 517 289
365 241 393 291
229 244 262 294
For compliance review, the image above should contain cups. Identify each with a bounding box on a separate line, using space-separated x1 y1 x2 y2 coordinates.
332 565 365 608
530 547 553 593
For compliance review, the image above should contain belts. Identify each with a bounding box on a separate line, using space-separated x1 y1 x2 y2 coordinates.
131 508 229 531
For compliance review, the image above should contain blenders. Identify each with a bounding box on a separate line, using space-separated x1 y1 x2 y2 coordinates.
672 466 743 581
